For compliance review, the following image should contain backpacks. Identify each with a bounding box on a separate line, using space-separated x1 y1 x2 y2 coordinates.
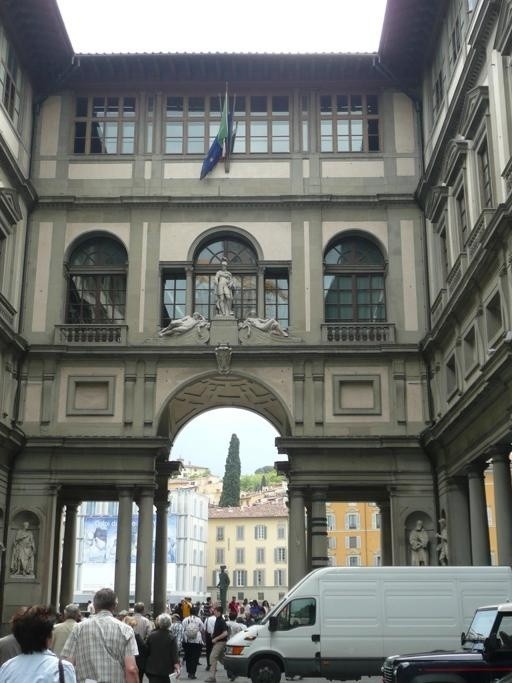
186 623 197 638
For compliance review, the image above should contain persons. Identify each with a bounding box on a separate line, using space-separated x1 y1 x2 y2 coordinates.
244 308 289 336
409 519 430 566
435 518 449 565
158 310 207 337
0 587 270 683
214 260 237 314
217 565 230 612
10 520 36 575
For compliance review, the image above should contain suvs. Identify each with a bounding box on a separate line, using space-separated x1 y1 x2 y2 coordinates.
380 603 510 683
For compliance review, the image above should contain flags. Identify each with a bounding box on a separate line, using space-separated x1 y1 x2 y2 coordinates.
200 89 228 179
228 96 236 155
231 120 238 151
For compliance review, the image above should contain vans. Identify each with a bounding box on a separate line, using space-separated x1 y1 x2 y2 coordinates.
222 567 511 683
